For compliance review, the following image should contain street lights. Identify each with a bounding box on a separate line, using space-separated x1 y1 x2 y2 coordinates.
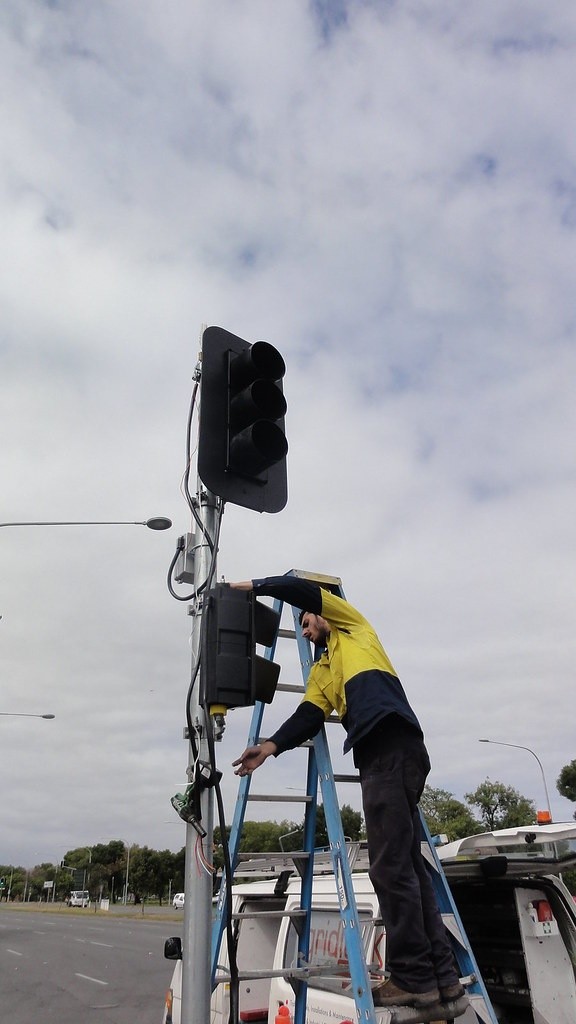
1 711 56 721
1 513 174 535
479 737 556 826
285 785 324 797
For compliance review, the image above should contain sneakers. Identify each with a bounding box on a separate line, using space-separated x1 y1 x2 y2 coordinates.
439 981 465 1002
371 979 441 1008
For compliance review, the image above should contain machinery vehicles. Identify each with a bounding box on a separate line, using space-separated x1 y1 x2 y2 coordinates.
162 827 576 1024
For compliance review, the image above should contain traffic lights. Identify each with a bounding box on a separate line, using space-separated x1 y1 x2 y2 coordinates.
197 327 293 516
204 581 282 714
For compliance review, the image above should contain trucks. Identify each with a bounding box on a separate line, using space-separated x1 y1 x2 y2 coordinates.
66 890 90 908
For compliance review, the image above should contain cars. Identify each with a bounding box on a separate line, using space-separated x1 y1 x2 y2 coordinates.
173 892 185 910
120 897 128 902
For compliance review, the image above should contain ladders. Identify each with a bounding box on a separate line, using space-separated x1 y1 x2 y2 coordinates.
211 568 498 1024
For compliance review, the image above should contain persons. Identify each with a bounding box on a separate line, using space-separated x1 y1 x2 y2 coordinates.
230 576 466 1009
